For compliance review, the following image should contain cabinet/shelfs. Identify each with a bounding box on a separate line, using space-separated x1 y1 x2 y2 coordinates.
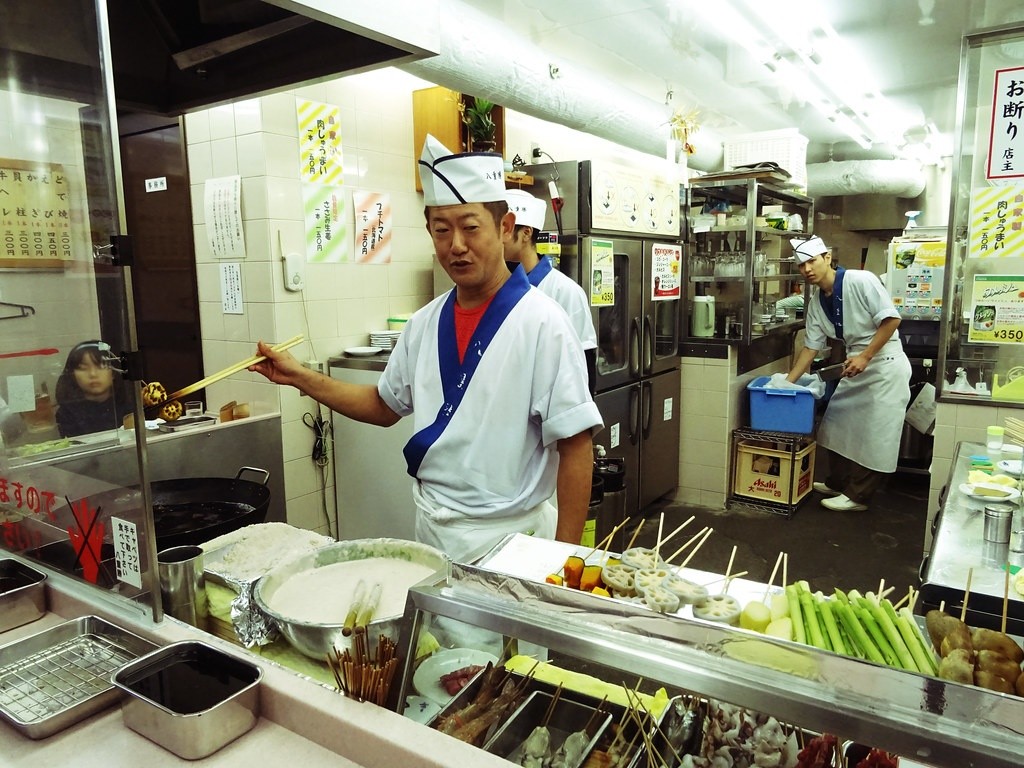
685 178 815 344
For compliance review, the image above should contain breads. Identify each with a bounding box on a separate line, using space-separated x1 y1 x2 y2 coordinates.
973 474 1018 497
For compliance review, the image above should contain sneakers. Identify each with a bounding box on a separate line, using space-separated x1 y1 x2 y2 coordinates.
821 494 868 512
813 482 842 496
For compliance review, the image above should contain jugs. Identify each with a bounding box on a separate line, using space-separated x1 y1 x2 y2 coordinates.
691 295 715 338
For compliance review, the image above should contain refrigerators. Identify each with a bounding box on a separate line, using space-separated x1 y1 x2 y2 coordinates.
508 160 684 516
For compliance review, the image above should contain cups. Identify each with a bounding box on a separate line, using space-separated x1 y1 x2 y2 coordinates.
987 425 1004 456
157 547 208 636
691 250 766 277
185 400 203 416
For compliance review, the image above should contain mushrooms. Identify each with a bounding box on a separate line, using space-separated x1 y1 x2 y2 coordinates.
926 610 1024 699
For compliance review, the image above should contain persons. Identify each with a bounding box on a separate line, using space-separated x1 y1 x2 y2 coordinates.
249 134 606 666
55 340 136 439
787 234 911 511
502 188 599 400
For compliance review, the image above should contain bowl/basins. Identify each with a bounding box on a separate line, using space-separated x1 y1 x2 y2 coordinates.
816 363 850 382
253 536 453 662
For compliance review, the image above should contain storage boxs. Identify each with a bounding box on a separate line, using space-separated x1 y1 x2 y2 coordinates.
724 128 810 190
747 376 815 433
734 440 817 504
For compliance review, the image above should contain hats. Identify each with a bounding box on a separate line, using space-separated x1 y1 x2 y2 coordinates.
417 133 506 207
790 233 828 266
504 188 547 231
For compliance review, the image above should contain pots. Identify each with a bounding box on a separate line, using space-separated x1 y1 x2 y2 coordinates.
35 467 270 565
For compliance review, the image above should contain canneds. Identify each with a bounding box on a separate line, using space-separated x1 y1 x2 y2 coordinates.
973 305 996 330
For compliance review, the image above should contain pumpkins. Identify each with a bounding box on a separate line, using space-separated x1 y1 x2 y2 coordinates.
546 555 623 599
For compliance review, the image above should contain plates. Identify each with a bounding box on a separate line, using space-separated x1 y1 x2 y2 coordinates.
998 459 1023 475
959 482 1019 502
344 347 382 356
1001 444 1022 453
370 331 401 353
403 695 442 725
412 647 503 701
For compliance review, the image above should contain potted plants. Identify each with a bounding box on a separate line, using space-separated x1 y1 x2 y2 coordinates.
459 98 498 152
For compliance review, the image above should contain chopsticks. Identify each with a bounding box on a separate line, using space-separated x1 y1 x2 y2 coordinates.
144 333 306 414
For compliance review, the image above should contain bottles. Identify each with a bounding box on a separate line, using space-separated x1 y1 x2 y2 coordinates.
984 504 1013 545
